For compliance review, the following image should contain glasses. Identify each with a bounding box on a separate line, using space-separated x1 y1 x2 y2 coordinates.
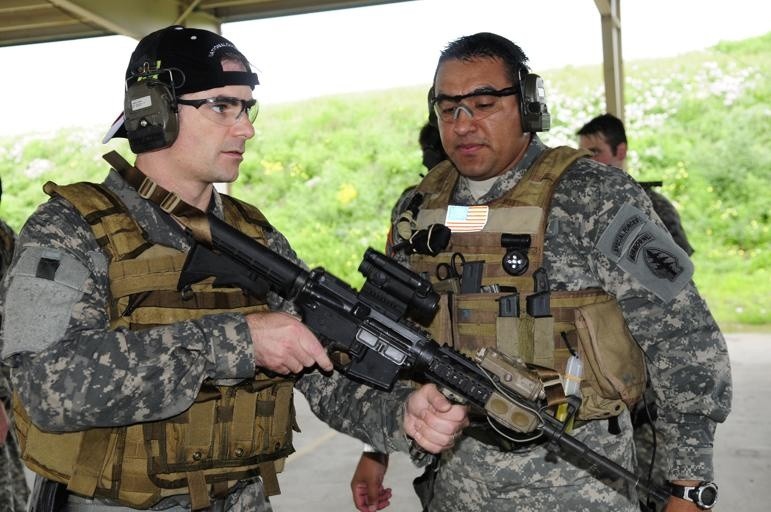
178 97 260 126
430 87 518 123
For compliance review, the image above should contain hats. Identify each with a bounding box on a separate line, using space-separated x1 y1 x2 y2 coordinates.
102 24 260 144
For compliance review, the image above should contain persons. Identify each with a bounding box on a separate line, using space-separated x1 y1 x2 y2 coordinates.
0 23 475 512
1 171 40 512
578 110 695 269
348 26 735 511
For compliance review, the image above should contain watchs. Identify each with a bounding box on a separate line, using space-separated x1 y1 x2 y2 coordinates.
666 477 722 510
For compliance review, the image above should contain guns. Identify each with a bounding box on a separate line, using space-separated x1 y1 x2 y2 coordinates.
177 209 669 502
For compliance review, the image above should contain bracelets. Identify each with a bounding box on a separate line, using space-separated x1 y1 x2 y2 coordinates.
363 444 391 467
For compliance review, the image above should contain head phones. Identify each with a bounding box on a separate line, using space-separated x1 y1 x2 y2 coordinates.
428 34 550 132
124 24 184 152
